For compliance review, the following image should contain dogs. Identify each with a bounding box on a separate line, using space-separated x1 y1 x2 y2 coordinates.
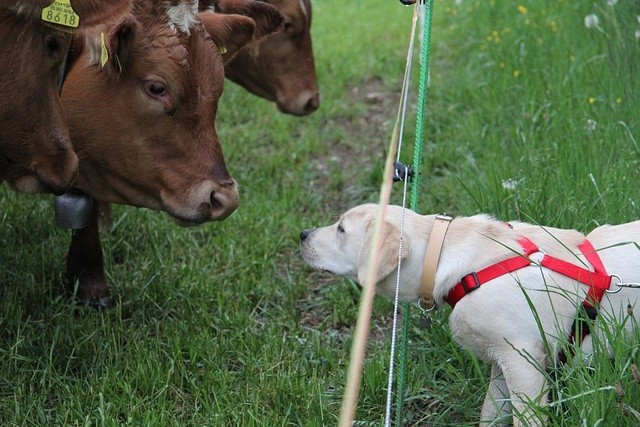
297 203 640 427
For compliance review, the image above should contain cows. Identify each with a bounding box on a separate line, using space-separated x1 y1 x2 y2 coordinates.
0 1 82 199
0 0 257 230
52 0 322 311
